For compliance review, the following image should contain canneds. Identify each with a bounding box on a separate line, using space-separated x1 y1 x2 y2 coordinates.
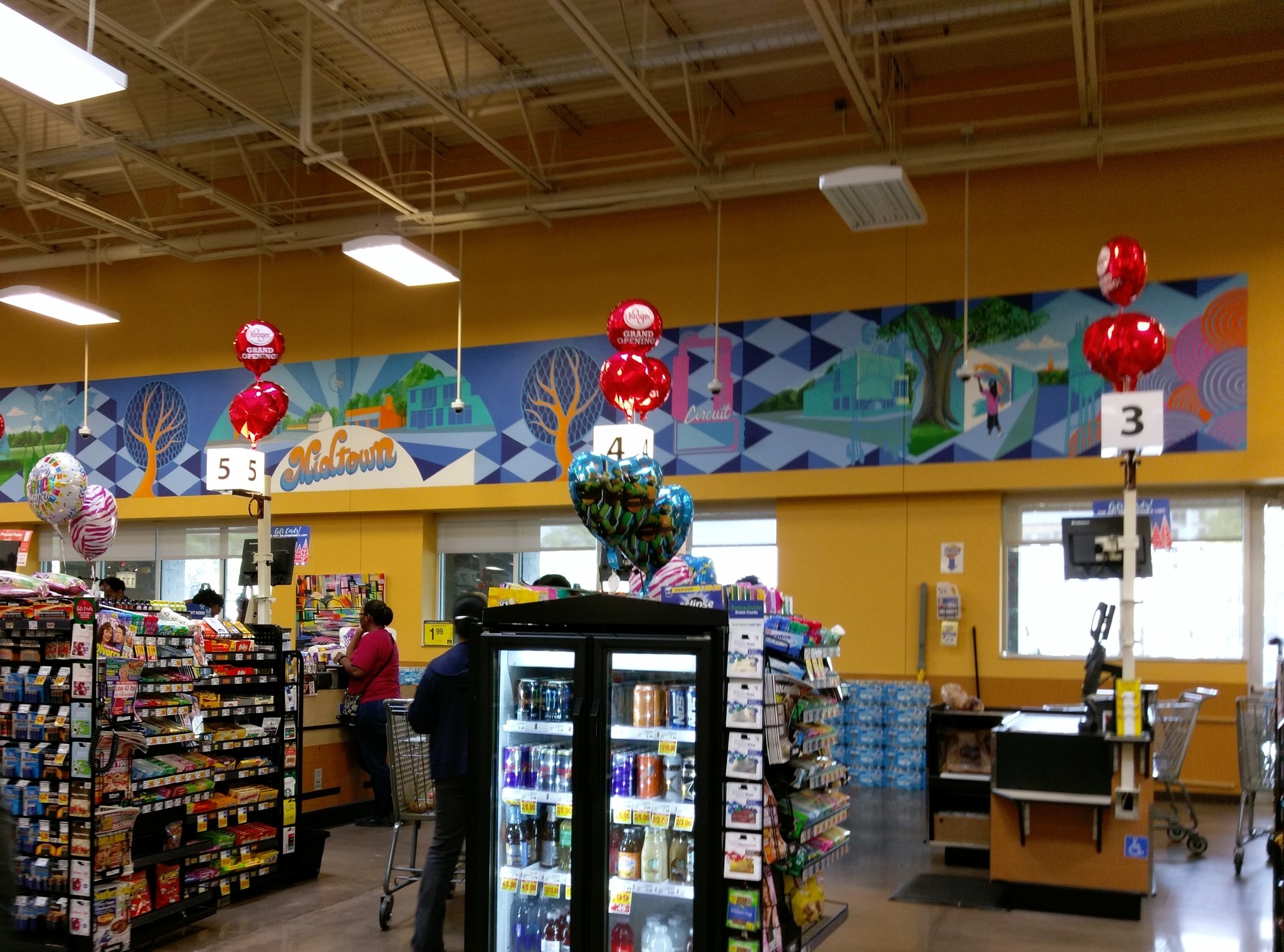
608 680 696 729
610 744 695 802
503 741 572 793
516 676 574 722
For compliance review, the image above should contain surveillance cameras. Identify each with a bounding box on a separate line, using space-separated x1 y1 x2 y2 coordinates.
707 379 723 394
79 426 91 439
451 399 465 413
956 364 972 382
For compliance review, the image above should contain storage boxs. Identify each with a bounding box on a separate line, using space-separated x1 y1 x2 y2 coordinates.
235 758 261 769
209 664 253 676
260 787 273 792
203 722 246 741
219 863 246 876
219 698 238 707
252 784 264 788
229 696 252 706
191 693 221 709
257 789 279 801
249 694 274 705
227 785 253 799
255 786 268 789
242 859 266 871
233 788 260 805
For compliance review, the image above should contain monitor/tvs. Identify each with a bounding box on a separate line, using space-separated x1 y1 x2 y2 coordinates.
1080 643 1105 697
238 537 296 586
1061 514 1153 579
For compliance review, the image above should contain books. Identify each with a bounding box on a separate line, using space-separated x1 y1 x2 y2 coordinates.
88 603 140 952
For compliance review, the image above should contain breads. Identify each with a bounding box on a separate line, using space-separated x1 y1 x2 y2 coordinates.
407 799 436 813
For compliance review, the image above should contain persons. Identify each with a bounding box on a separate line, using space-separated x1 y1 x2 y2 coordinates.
531 573 571 589
98 576 131 601
196 626 202 634
192 637 205 667
332 600 411 827
191 588 224 619
97 622 114 644
124 635 133 648
406 592 492 952
112 624 126 657
110 664 130 714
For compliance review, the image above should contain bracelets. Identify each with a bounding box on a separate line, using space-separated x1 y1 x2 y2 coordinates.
338 655 347 664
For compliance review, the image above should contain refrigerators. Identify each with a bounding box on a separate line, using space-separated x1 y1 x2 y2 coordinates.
472 592 730 952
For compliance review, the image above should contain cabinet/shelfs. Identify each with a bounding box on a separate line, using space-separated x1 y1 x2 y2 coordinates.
0 594 304 952
722 611 853 952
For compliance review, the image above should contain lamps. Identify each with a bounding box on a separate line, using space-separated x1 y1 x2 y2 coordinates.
0 0 128 106
0 228 120 325
818 163 930 233
340 229 463 288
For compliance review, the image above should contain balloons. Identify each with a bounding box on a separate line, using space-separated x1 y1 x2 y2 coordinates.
0 413 5 441
68 484 118 560
1080 238 1167 392
616 483 695 581
598 299 673 422
567 451 665 554
228 321 288 443
26 452 87 540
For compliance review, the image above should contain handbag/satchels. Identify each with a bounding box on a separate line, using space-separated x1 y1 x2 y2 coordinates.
340 692 361 726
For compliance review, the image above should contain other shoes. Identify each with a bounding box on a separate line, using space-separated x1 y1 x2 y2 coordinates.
356 816 395 827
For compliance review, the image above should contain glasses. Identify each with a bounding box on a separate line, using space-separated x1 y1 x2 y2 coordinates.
357 612 367 617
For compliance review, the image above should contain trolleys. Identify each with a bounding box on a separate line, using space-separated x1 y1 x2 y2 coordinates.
378 698 466 931
1151 685 1220 856
1231 685 1276 876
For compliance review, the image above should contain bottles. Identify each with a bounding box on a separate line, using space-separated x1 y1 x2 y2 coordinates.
510 891 570 952
100 597 187 612
611 916 634 952
640 902 693 952
500 800 572 874
609 821 693 886
823 679 931 789
